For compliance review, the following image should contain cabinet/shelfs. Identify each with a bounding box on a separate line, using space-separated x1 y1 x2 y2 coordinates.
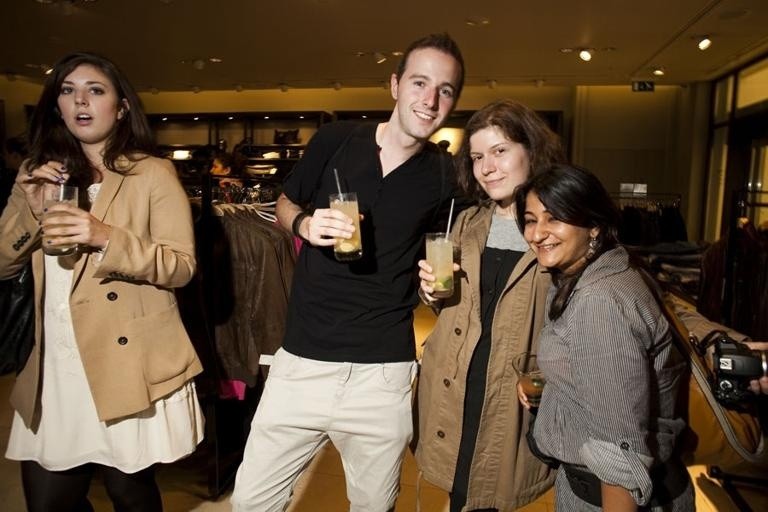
140 110 564 210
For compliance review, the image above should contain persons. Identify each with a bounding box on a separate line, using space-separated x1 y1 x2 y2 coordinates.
208 152 252 190
228 29 480 512
407 100 766 512
1 137 29 216
1 51 208 511
514 161 698 512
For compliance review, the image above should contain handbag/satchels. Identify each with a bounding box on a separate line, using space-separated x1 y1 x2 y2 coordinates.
274 127 299 144
0 263 35 380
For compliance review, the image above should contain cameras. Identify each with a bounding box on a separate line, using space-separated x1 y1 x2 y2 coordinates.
712 337 762 414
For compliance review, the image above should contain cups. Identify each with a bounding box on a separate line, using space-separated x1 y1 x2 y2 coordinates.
329 193 363 262
42 186 78 256
425 232 454 299
511 352 546 408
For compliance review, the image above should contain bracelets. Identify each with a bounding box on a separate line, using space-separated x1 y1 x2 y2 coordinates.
292 212 310 236
